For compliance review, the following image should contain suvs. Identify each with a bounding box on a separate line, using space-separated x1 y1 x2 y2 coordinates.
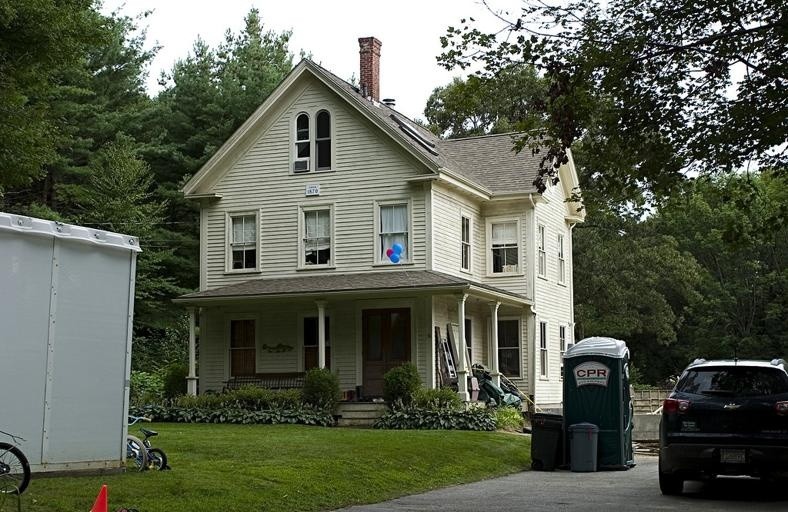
657 353 788 500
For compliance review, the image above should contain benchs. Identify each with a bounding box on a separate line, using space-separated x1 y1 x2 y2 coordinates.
221 371 306 394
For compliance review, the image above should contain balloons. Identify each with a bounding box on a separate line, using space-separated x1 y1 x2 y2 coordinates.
392 243 403 255
386 248 394 256
390 253 400 263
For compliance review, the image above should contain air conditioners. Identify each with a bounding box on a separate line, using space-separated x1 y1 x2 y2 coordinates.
292 157 309 173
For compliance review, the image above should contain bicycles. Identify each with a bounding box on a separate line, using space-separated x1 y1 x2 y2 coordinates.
0 441 33 497
126 435 148 474
128 414 169 471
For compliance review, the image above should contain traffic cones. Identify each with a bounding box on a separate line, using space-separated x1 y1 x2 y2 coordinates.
89 483 108 512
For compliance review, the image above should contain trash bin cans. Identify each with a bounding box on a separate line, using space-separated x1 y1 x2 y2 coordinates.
568 422 599 472
530 413 566 472
